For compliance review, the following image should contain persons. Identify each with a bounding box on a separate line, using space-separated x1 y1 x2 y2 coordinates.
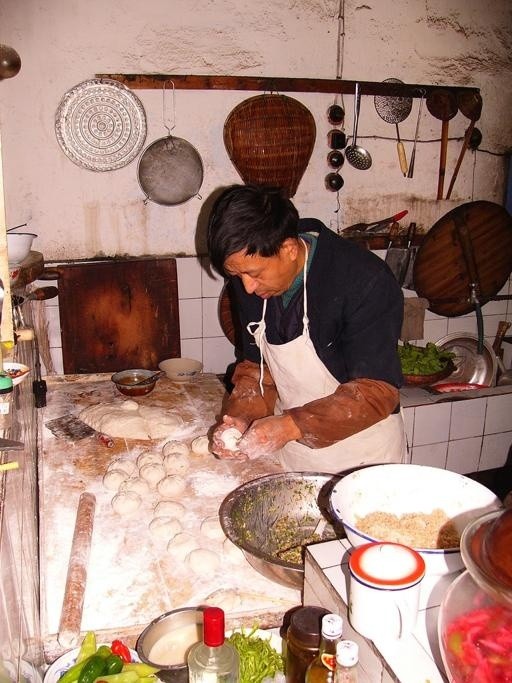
209 186 408 474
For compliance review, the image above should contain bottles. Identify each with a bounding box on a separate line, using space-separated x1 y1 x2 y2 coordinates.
0 373 12 429
188 607 240 683
306 613 343 682
333 640 359 682
32 362 48 407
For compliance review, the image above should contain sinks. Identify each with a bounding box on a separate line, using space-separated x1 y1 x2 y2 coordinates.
398 336 512 476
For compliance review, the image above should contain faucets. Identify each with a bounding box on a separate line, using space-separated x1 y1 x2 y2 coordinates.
459 282 484 355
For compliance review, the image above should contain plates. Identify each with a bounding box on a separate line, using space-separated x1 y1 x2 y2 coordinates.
4 657 44 682
221 625 288 682
42 643 159 683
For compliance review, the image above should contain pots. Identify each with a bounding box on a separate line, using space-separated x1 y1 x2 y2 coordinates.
0 284 59 332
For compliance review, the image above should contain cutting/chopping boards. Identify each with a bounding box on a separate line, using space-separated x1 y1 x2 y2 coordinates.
54 257 183 374
0 250 44 290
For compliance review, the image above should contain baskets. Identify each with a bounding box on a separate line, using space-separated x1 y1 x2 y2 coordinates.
222 94 316 197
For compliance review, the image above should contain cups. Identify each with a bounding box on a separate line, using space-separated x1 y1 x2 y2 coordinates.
347 540 427 640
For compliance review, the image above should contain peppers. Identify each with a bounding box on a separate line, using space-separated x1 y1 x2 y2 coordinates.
57 631 160 682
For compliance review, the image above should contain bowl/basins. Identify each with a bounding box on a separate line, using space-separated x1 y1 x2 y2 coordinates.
109 368 159 396
5 263 23 284
159 357 203 384
138 605 206 682
429 381 491 394
6 232 37 262
218 471 348 591
3 362 31 386
329 463 506 576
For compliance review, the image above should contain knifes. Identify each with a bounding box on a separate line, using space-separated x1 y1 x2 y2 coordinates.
382 221 411 287
42 410 114 448
402 221 422 291
